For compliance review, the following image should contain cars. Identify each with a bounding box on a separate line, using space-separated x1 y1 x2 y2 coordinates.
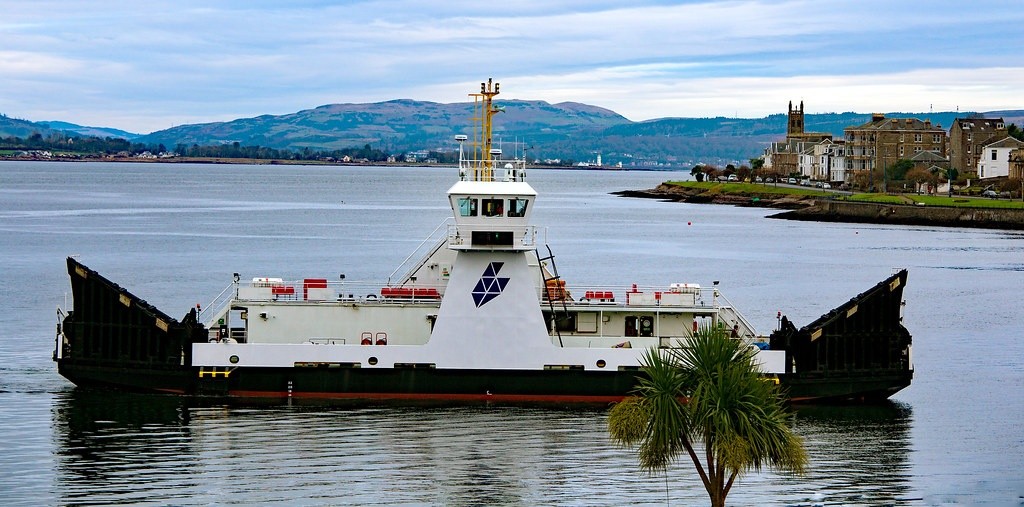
822 182 831 189
999 191 1011 199
815 182 823 188
838 183 850 192
718 173 816 187
983 190 998 199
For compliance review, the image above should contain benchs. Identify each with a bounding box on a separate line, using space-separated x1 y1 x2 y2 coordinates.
272 287 294 298
586 291 614 299
382 288 439 300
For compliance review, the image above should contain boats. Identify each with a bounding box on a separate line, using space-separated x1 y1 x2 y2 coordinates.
51 78 915 410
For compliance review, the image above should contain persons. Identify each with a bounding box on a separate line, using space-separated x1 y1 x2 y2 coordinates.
730 325 743 343
